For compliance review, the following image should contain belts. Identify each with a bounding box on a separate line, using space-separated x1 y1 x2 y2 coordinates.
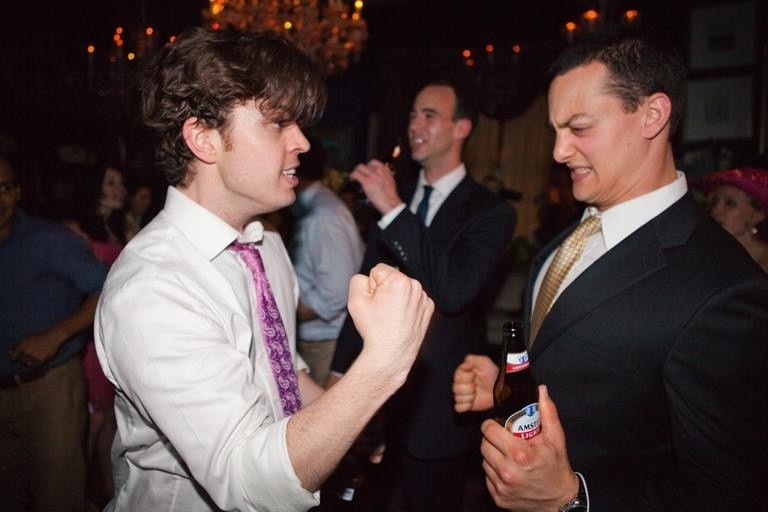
1 369 47 389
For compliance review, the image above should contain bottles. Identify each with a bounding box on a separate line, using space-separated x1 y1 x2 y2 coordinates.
354 142 404 212
493 318 542 447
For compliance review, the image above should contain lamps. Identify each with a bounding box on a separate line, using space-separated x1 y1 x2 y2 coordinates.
205 0 369 77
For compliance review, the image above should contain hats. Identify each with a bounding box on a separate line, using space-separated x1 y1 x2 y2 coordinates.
686 166 768 211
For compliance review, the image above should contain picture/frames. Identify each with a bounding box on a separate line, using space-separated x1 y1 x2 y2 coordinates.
677 69 758 147
686 2 761 75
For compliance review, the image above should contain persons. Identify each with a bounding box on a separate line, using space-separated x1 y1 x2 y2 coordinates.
686 165 767 274
90 25 438 512
345 77 518 512
482 174 519 311
533 162 587 251
0 138 368 512
449 37 767 512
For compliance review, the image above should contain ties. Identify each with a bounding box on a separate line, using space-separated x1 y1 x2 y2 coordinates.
228 243 303 415
417 186 433 225
529 216 602 351
